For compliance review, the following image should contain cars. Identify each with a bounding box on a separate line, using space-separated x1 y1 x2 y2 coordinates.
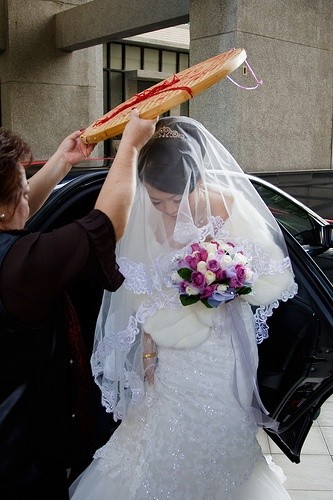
39 168 333 283
16 172 333 499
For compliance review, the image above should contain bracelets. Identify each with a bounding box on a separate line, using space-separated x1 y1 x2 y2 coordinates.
141 350 161 359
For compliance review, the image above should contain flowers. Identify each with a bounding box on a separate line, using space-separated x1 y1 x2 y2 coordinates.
169 238 256 310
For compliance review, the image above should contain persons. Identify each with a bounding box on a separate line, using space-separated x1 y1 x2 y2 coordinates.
2 104 163 500
58 113 292 498
